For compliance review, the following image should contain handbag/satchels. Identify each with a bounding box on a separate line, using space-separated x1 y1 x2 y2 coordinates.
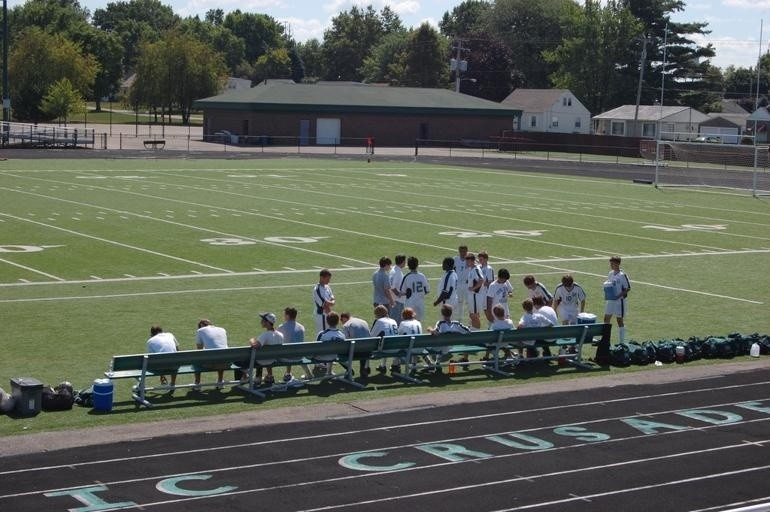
607 331 770 368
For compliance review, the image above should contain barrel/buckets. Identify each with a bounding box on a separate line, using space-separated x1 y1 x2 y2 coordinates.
577 312 597 343
749 342 760 357
92 378 114 412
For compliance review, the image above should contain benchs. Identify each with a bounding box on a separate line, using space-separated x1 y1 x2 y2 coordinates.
103 321 612 409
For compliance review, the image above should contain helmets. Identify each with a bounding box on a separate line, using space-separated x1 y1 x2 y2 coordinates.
522 298 533 311
400 307 416 320
326 310 340 326
373 304 388 318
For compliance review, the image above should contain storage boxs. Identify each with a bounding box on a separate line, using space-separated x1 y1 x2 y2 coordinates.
91 376 116 413
11 375 45 418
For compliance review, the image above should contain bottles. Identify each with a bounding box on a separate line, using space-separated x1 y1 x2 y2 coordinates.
750 342 761 358
675 345 684 364
449 360 456 377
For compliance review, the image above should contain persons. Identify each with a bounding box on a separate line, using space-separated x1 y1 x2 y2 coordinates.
131 325 181 393
400 256 432 323
603 254 632 344
262 305 305 383
390 307 423 371
363 304 398 374
189 318 229 390
339 311 372 379
427 243 587 374
313 267 336 375
388 253 407 326
371 255 396 316
238 311 284 385
298 310 346 380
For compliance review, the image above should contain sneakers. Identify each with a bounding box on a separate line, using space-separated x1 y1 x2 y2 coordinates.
190 383 201 391
375 365 387 371
343 368 355 379
282 373 292 381
359 366 371 374
390 344 580 376
215 379 225 389
264 375 274 384
168 382 175 390
316 361 337 377
254 376 262 385
591 339 600 347
160 376 167 384
301 373 315 380
239 374 251 385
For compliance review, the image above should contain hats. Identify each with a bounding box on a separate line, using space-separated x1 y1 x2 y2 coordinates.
258 310 277 324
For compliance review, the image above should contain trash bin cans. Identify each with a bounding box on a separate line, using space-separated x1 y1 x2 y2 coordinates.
231 135 239 144
